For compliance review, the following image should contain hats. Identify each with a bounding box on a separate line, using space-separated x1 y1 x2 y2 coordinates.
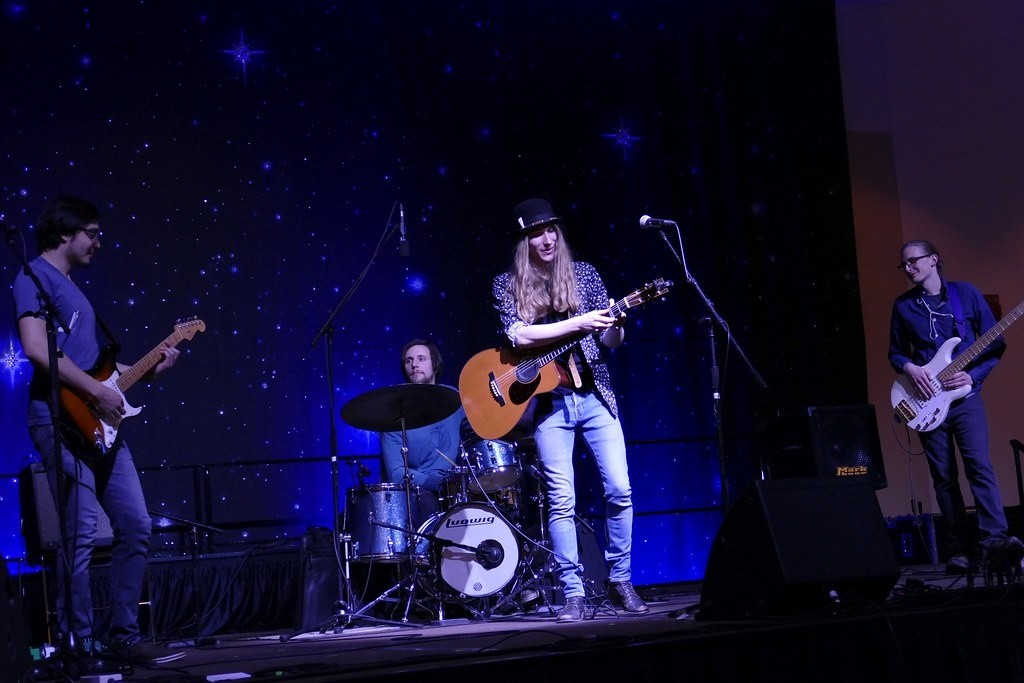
507 199 565 241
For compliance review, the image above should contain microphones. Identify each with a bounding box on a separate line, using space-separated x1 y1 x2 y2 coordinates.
357 461 373 477
484 546 502 563
640 215 676 228
399 200 409 256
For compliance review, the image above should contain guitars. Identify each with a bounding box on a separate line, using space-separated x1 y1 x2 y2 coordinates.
458 276 674 440
48 316 206 460
890 301 1024 432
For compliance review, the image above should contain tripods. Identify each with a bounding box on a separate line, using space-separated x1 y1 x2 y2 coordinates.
279 223 607 641
0 223 224 683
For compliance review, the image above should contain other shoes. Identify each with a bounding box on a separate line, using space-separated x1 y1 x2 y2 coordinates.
74 640 121 663
989 535 1024 570
945 553 971 574
120 639 186 663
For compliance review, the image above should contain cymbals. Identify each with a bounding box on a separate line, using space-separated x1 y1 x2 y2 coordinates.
340 384 463 432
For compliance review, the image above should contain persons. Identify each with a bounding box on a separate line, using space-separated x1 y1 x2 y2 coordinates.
379 339 544 603
490 198 650 623
10 196 188 675
887 239 1024 576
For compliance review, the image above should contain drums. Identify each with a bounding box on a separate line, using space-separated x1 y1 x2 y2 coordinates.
436 476 519 512
459 439 523 495
408 501 520 604
345 485 431 565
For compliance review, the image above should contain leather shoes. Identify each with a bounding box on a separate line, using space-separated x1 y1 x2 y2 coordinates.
556 596 585 622
607 581 649 614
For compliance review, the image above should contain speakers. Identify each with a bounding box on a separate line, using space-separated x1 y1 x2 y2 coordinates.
752 404 888 491
20 463 116 559
696 474 901 620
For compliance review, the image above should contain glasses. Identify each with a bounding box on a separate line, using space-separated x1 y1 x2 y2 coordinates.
80 227 104 242
897 254 930 271
